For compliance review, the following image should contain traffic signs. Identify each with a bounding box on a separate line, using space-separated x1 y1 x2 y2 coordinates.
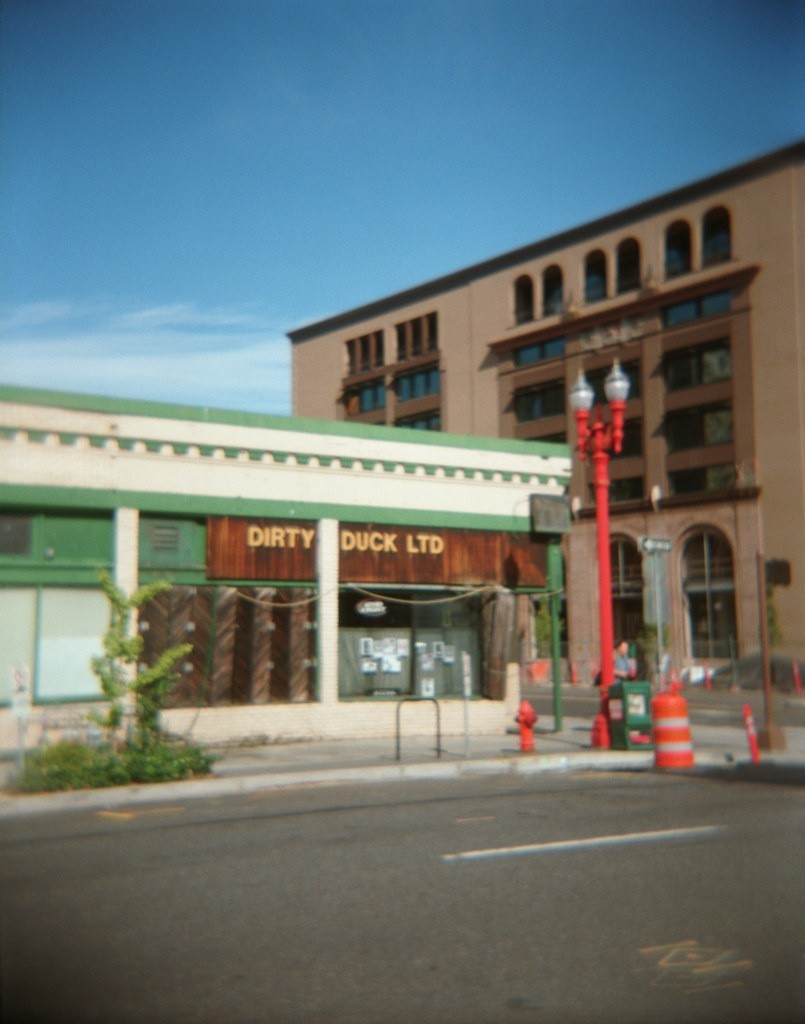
639 537 672 556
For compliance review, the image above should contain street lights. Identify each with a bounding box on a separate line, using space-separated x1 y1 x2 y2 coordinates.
570 357 630 750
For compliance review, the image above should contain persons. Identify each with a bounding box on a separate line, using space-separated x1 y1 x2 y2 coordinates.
612 640 631 685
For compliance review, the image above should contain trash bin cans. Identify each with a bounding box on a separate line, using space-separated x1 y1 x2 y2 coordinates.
607 680 654 750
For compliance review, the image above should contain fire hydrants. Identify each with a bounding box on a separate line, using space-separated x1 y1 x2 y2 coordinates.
514 700 538 754
